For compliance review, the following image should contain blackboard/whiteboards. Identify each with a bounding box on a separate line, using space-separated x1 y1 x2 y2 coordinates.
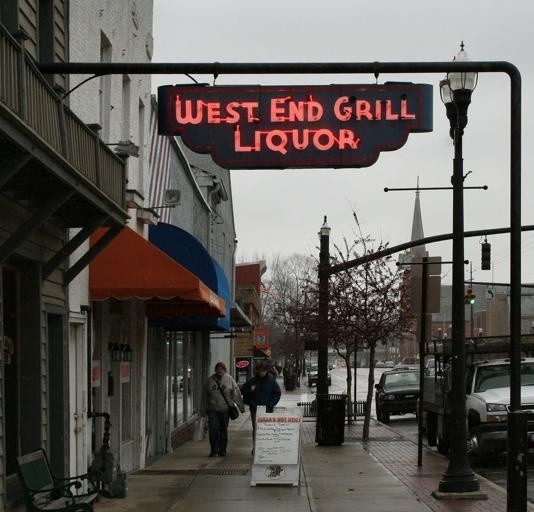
254 416 302 479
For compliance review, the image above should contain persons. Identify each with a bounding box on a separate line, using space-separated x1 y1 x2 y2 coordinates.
197 362 246 458
237 360 281 457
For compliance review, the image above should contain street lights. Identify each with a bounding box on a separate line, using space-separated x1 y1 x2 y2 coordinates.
440 41 479 478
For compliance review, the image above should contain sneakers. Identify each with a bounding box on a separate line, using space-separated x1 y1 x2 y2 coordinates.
209 451 217 457
218 451 227 457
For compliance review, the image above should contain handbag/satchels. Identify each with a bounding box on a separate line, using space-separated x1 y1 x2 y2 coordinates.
212 376 240 421
192 417 206 442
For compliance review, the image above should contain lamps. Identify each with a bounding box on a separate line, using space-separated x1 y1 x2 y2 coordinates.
153 189 181 209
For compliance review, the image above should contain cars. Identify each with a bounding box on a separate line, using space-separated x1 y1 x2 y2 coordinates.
375 356 534 470
305 364 336 387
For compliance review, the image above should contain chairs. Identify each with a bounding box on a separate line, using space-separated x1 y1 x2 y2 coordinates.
16 448 101 512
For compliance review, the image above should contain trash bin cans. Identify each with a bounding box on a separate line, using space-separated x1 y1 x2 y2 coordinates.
315 394 349 446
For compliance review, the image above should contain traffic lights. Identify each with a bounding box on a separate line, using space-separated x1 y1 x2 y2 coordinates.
465 288 476 305
482 243 491 269
